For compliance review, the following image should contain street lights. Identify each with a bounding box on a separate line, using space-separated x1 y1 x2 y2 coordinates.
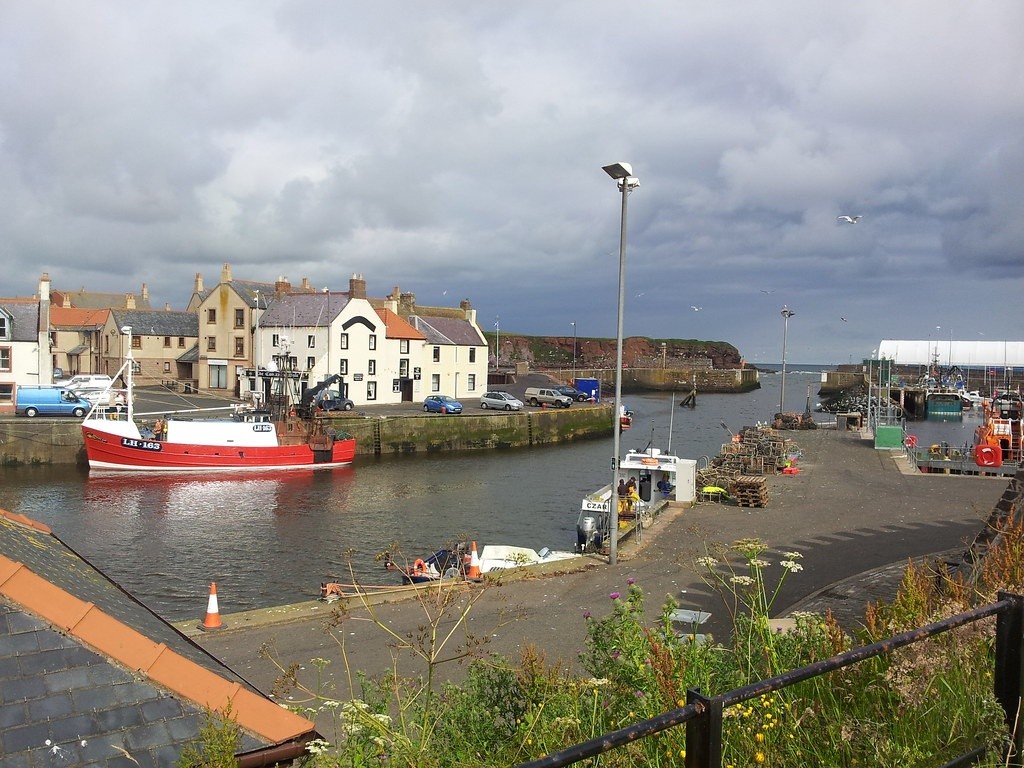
780 305 797 415
600 160 641 565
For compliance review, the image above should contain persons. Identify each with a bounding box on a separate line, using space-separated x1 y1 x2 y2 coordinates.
626 477 636 510
109 392 117 420
323 393 330 400
154 416 167 442
618 479 629 511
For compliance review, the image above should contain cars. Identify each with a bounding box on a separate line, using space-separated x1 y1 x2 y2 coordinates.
552 384 588 402
56 373 128 412
423 395 463 414
14 385 91 417
525 386 573 409
316 391 354 411
480 391 523 411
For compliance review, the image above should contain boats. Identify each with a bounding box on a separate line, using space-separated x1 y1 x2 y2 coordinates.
404 543 580 585
578 447 677 549
80 418 357 475
914 366 976 409
974 388 1023 462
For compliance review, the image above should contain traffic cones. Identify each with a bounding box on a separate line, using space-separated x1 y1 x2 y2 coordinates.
200 578 229 633
466 540 485 582
